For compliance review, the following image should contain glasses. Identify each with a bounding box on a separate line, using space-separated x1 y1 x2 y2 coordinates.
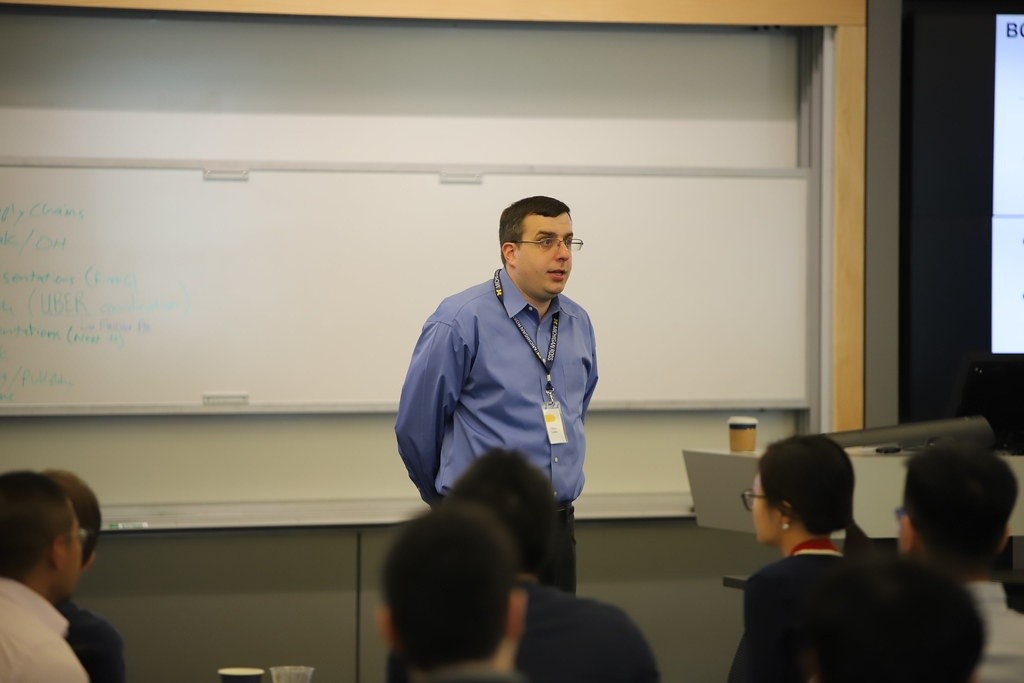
58 528 88 542
511 237 583 255
742 489 766 510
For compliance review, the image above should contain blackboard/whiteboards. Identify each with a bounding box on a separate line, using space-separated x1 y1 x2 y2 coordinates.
0 22 822 531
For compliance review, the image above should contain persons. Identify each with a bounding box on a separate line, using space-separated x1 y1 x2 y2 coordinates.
375 500 532 683
447 448 662 683
396 196 598 596
42 469 125 683
728 434 877 683
0 470 90 683
897 443 1024 683
799 554 988 683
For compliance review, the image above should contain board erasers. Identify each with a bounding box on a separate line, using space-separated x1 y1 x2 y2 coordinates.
203 395 249 406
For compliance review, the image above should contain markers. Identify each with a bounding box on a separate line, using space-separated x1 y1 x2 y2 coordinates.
109 522 149 530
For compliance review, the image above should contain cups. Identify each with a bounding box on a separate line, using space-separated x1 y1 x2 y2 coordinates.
269 665 315 683
728 416 758 453
216 667 264 683
